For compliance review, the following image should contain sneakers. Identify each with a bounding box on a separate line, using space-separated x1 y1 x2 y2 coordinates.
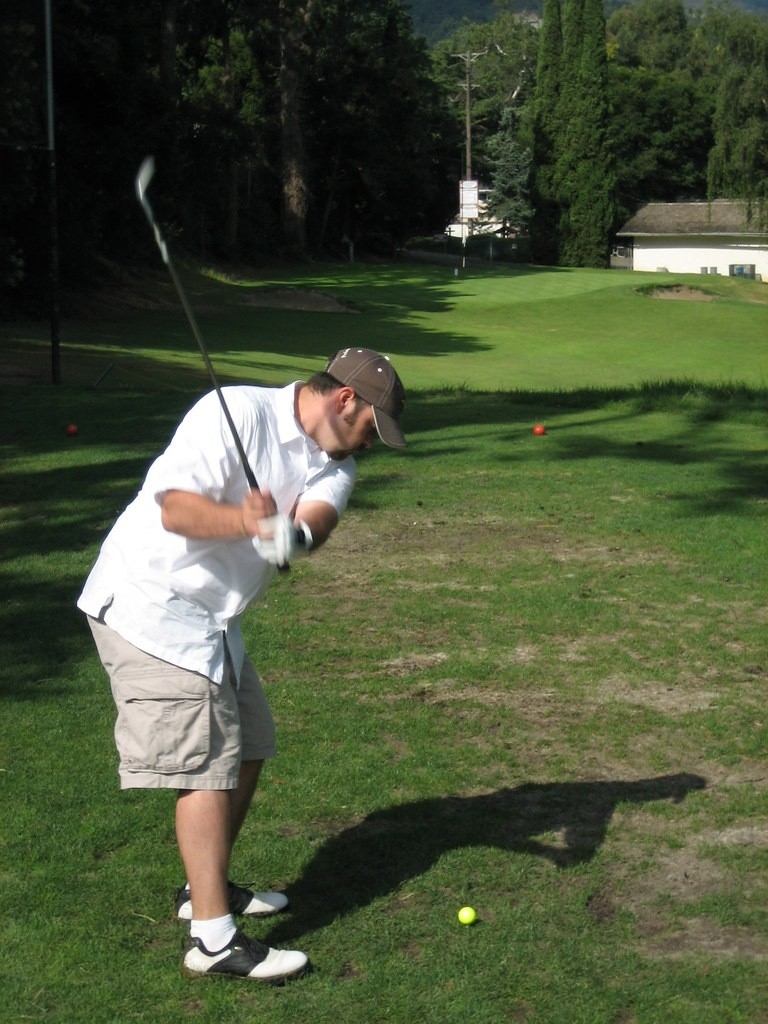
173 877 290 921
179 923 310 981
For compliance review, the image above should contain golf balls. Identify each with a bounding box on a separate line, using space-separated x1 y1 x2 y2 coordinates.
457 906 477 925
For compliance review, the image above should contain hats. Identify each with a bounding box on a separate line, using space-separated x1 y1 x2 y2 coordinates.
326 347 409 448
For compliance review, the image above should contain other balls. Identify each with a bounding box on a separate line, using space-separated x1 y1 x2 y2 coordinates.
533 423 545 436
66 423 79 436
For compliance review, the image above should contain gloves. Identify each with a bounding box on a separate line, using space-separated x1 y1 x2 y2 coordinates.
251 516 313 565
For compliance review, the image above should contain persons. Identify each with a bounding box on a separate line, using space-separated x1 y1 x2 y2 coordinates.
77 349 406 982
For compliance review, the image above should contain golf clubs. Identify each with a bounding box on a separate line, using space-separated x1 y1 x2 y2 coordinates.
132 154 292 575
